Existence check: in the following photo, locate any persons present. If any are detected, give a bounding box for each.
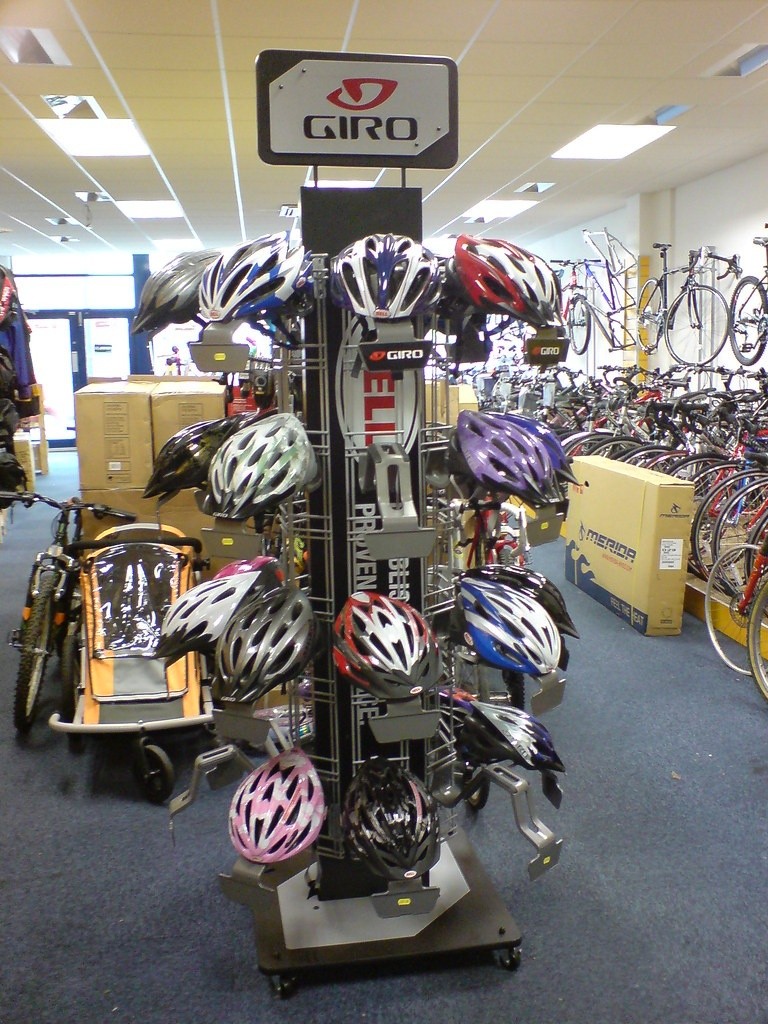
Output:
[163,346,185,377]
[165,358,175,376]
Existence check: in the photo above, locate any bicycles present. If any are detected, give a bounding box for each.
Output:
[462,233,768,703]
[0,489,140,756]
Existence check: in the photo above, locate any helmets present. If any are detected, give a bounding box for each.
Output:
[451,409,555,498]
[437,689,478,728]
[329,233,443,322]
[332,591,443,699]
[426,255,488,338]
[130,249,223,331]
[486,411,579,488]
[215,584,320,705]
[453,234,564,330]
[213,555,281,585]
[470,701,568,773]
[228,747,326,864]
[460,575,561,675]
[464,563,580,640]
[336,755,440,879]
[206,412,319,521]
[198,231,315,323]
[141,412,246,507]
[149,570,264,668]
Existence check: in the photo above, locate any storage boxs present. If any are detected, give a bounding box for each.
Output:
[80,489,158,561]
[425,379,459,434]
[73,381,158,491]
[159,489,257,582]
[13,431,35,492]
[459,383,479,411]
[565,455,695,638]
[151,381,226,489]
[227,386,274,416]
